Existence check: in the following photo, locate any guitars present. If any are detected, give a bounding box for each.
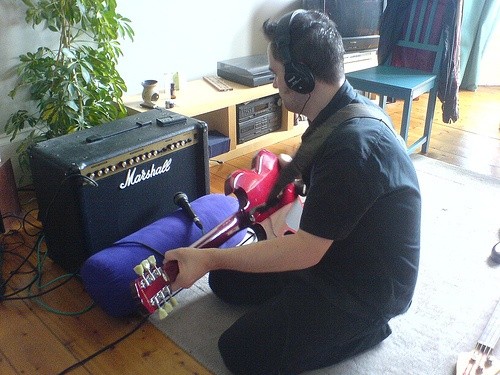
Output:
[129,149,303,320]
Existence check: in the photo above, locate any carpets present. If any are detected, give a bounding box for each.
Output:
[148,152,500,374]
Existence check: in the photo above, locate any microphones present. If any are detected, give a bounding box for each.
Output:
[174,191,205,231]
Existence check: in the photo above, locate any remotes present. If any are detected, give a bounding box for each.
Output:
[156,114,187,127]
[202,74,233,91]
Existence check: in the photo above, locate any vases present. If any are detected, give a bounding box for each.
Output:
[140,80,161,107]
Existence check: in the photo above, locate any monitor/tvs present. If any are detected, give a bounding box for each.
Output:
[301,0,387,51]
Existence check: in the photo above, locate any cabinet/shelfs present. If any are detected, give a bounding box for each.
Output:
[118,47,380,170]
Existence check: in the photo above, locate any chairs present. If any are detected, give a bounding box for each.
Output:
[342,1,465,160]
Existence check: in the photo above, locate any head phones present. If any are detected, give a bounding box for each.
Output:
[274,8,317,94]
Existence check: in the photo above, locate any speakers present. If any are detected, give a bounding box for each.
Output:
[29,106,212,273]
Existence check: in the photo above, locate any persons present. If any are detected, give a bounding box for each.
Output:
[162,9,421,373]
